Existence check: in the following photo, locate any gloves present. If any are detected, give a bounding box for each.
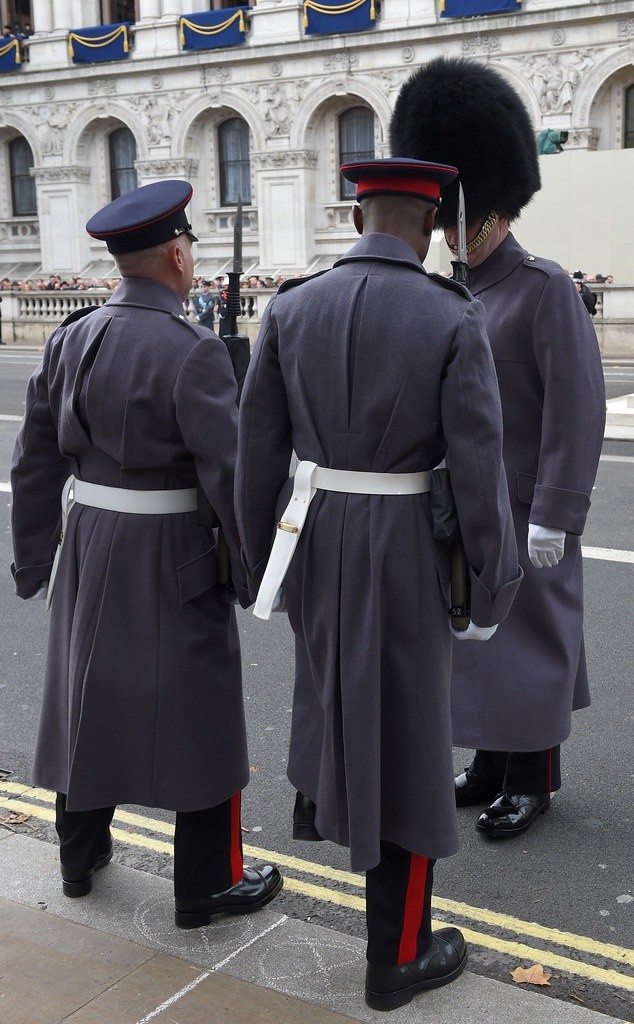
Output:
[32,581,48,599]
[271,588,287,614]
[527,523,566,569]
[448,618,500,642]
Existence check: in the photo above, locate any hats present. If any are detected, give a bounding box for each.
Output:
[86,179,199,253]
[201,281,212,287]
[338,156,460,209]
[388,53,540,228]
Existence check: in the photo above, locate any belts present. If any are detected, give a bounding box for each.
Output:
[41,473,196,614]
[253,452,435,620]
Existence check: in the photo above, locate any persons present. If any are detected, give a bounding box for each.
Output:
[0,274,122,316]
[0,296,6,346]
[185,275,288,318]
[192,280,215,332]
[217,288,229,340]
[435,165,608,843]
[0,20,34,64]
[10,179,284,929]
[231,155,526,1014]
[565,270,614,319]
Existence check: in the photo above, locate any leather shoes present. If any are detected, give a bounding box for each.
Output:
[173,863,284,929]
[60,833,114,897]
[292,789,322,842]
[475,792,552,838]
[453,767,499,808]
[365,927,471,1010]
